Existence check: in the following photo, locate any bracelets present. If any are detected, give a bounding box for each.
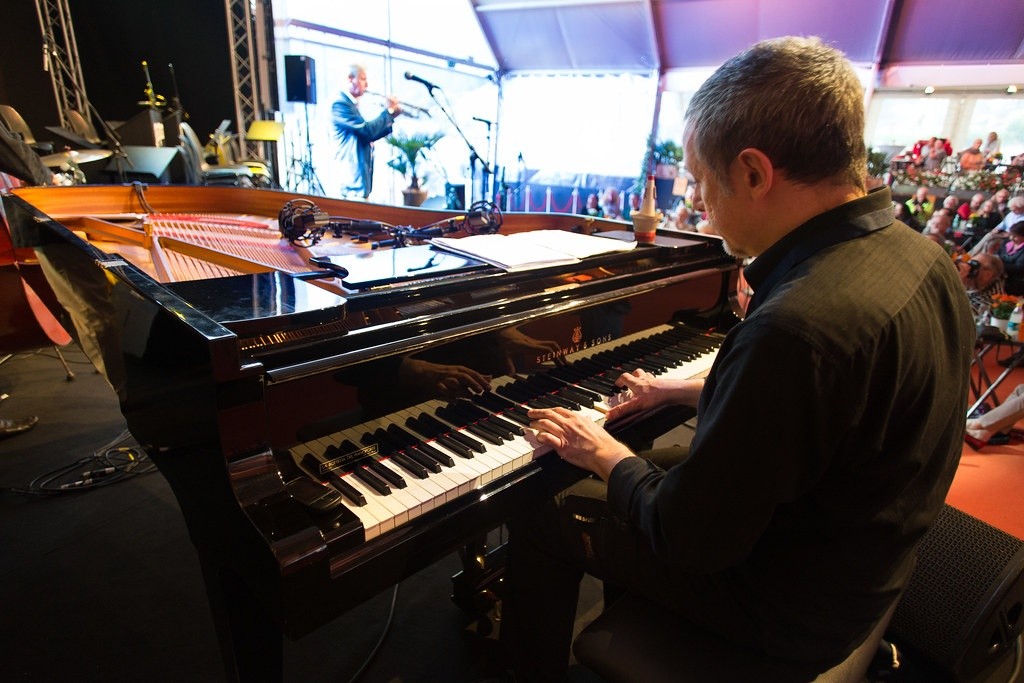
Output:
[388,107,394,115]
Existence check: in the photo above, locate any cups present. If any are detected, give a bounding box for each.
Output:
[631,214,658,243]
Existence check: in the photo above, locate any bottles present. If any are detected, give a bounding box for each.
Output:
[643,176,656,213]
[1006,303,1023,336]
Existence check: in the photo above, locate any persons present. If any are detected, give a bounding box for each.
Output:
[581,186,723,236]
[492,32,976,683]
[866,132,1024,450]
[328,64,403,202]
[403,328,570,398]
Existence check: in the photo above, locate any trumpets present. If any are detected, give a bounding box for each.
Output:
[366,90,431,119]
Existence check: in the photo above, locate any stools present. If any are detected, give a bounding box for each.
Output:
[572,557,920,683]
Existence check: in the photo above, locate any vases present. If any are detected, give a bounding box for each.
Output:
[990,316,1010,340]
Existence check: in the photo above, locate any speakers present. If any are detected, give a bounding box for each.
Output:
[285,54,317,104]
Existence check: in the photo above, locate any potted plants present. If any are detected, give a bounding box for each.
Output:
[865,147,891,192]
[647,139,683,181]
[383,130,446,207]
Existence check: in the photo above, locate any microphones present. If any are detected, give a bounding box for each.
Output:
[82,465,126,478]
[60,477,102,489]
[473,117,493,124]
[518,152,522,162]
[43,34,49,71]
[404,71,440,89]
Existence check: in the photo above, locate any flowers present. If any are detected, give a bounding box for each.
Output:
[892,163,1024,194]
[991,295,1018,320]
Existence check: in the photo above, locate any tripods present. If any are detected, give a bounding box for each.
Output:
[294,103,326,196]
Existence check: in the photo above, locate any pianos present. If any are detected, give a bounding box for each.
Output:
[0,179,748,683]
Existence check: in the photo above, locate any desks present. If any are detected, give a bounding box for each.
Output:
[966,334,1023,419]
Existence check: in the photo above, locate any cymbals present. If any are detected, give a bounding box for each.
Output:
[38,149,114,168]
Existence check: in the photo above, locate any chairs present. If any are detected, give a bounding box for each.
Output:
[175,122,273,190]
